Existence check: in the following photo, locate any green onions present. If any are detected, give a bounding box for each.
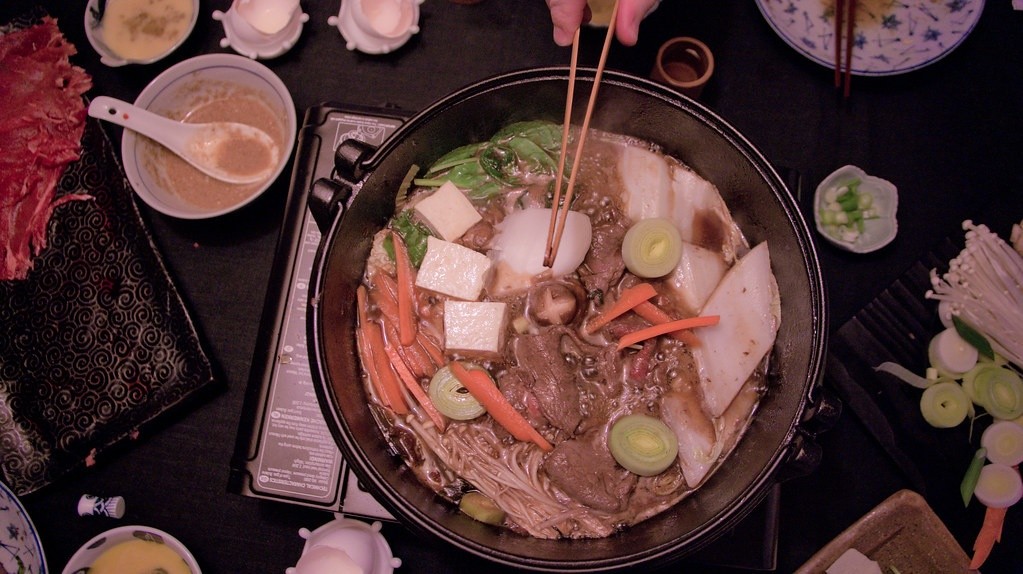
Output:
[872,327,1023,508]
[820,177,882,244]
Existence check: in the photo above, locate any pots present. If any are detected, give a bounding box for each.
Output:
[309,64,830,574]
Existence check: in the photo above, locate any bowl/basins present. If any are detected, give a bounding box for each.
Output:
[122,52,298,220]
[814,165,898,254]
[84,0,200,68]
[61,524,202,574]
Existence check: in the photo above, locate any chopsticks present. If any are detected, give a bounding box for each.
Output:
[542,0,620,268]
[831,0,855,96]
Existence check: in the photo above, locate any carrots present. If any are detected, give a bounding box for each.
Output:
[969,506,1007,569]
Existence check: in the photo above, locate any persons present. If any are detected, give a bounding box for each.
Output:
[546,0,1023,44]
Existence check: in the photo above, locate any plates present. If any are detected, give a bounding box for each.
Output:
[756,0,985,76]
[0,482,48,574]
[0,96,216,497]
[795,489,980,574]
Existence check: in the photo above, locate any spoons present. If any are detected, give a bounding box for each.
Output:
[86,96,279,184]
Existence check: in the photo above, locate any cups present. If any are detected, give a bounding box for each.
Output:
[651,37,715,100]
